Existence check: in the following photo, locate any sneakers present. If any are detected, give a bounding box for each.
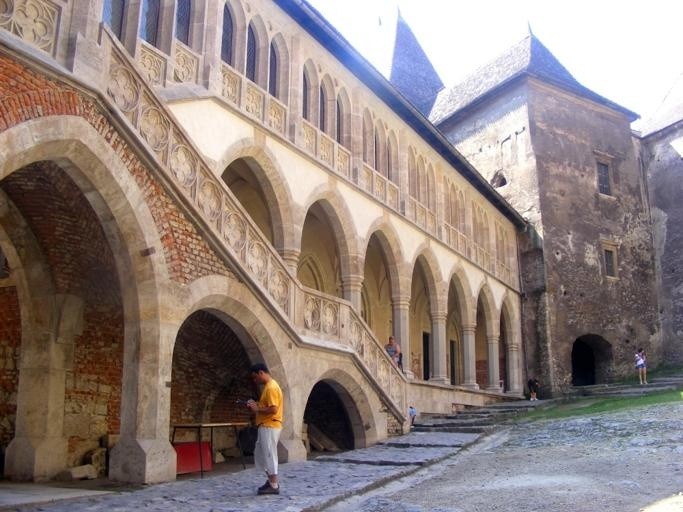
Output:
[258,481,279,495]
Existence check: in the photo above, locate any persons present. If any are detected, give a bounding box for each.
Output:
[528,376,538,401]
[633,348,648,385]
[247,364,282,494]
[384,336,400,366]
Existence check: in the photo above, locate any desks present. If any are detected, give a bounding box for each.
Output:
[171,421,252,477]
